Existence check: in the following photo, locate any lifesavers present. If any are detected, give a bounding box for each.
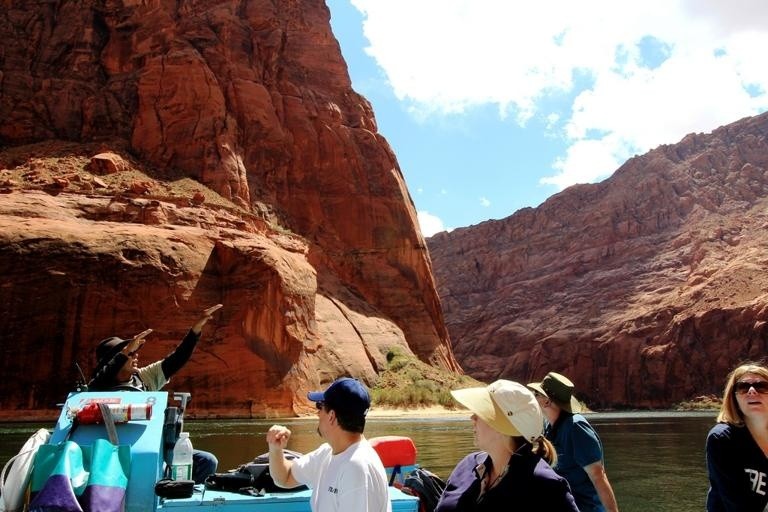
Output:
[0,427,51,510]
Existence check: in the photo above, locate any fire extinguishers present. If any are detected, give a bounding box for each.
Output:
[65,399,153,424]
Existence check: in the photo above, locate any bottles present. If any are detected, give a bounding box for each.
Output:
[70,403,153,425]
[171,431,193,481]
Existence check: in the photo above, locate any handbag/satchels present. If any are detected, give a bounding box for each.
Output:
[28,439,132,512]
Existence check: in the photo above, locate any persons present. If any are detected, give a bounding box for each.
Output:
[433,378,580,512]
[526,372,620,512]
[706,364,768,512]
[88,303,224,483]
[266,377,392,512]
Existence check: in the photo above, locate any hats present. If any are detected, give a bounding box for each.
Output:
[308,377,370,417]
[89,337,144,378]
[527,372,582,414]
[450,378,543,444]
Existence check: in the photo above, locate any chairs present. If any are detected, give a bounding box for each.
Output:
[369,437,419,486]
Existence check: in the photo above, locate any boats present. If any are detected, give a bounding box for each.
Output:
[0,389,426,512]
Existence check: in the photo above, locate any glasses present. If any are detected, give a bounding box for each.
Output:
[316,402,327,410]
[734,381,768,395]
